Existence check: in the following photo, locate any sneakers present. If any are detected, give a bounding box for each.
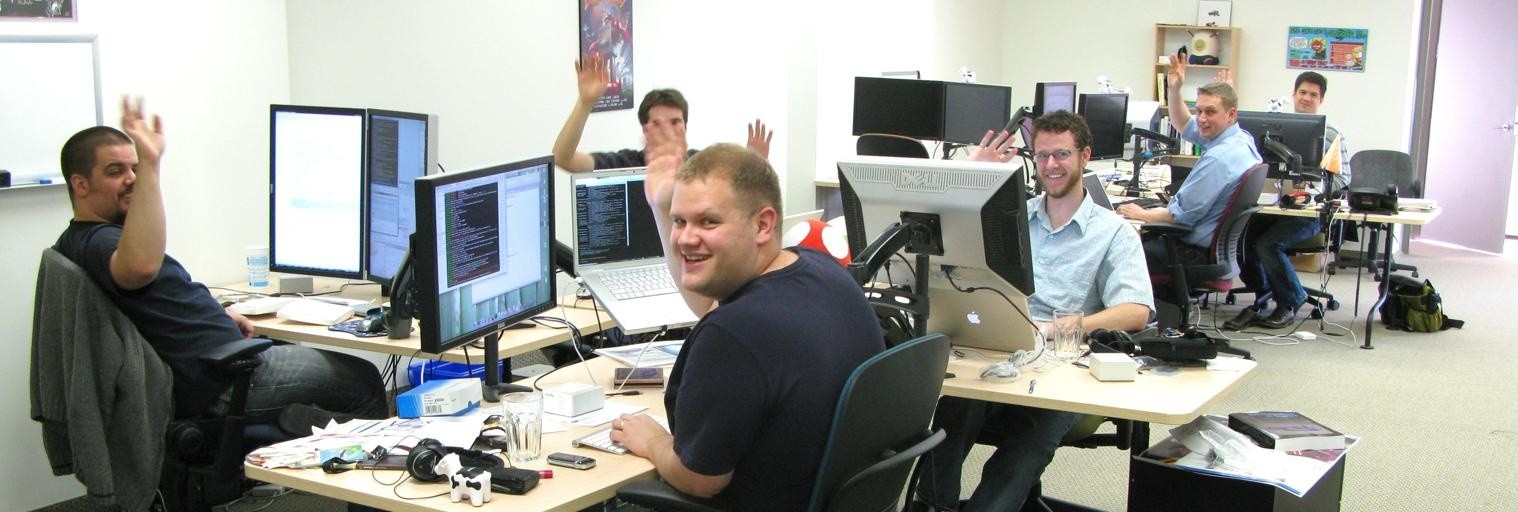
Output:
[278,402,378,436]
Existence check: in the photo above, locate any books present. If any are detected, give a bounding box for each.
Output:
[1228,411,1346,452]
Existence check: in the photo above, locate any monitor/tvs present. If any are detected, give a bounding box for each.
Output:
[1078,93,1129,161]
[414,154,558,403]
[269,104,367,280]
[944,82,1012,146]
[1117,101,1161,162]
[1236,111,1326,182]
[365,107,440,287]
[1033,81,1076,119]
[835,155,1036,296]
[572,413,671,456]
[852,76,945,141]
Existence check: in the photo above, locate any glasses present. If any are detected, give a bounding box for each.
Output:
[1032,147,1084,163]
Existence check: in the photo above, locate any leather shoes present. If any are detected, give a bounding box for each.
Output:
[1223,306,1262,331]
[1259,304,1294,328]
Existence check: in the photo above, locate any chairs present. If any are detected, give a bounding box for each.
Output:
[1328,150,1423,281]
[857,133,929,158]
[1143,163,1270,335]
[936,393,1134,510]
[29,249,289,512]
[616,334,951,512]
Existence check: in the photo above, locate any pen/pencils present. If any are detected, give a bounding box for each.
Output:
[538,470,553,479]
[1029,379,1036,393]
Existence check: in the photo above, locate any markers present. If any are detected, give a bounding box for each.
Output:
[40,178,67,184]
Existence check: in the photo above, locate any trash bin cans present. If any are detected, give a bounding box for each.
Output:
[407,359,504,388]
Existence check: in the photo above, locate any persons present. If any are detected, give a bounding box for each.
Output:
[913,108,1159,512]
[1114,52,1265,277]
[592,142,887,512]
[52,93,384,512]
[551,51,774,172]
[1223,71,1352,331]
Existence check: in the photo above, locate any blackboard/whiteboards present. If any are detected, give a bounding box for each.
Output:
[0,36,104,190]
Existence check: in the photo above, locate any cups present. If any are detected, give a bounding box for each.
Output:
[246,246,269,289]
[504,389,543,463]
[1052,307,1084,357]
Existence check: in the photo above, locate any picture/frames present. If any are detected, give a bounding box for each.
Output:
[1197,0,1232,26]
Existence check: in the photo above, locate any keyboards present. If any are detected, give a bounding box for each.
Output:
[1257,192,1278,205]
[1112,198,1163,211]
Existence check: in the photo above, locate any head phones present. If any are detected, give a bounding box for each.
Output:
[406,438,505,483]
[1089,328,1135,354]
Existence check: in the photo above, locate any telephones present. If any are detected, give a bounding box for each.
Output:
[1347,184,1399,213]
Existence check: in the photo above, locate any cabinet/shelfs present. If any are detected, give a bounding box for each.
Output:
[1152,25,1240,110]
[1128,414,1346,512]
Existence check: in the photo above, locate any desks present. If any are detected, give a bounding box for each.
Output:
[244,339,685,512]
[814,173,1171,223]
[208,271,616,383]
[1106,164,1441,350]
[863,283,1257,456]
[556,290,602,310]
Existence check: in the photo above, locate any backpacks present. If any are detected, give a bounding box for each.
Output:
[1377,273,1444,333]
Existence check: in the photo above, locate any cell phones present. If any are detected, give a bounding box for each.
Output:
[547,453,596,470]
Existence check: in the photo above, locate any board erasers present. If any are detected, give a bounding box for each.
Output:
[0,169,11,188]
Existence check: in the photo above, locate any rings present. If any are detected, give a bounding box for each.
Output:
[621,419,625,429]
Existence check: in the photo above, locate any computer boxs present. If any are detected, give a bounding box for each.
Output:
[1127,436,1346,512]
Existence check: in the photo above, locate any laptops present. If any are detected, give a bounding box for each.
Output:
[570,166,700,337]
[925,288,1054,353]
[1082,171,1116,213]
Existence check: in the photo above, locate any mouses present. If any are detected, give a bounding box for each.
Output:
[357,315,386,333]
